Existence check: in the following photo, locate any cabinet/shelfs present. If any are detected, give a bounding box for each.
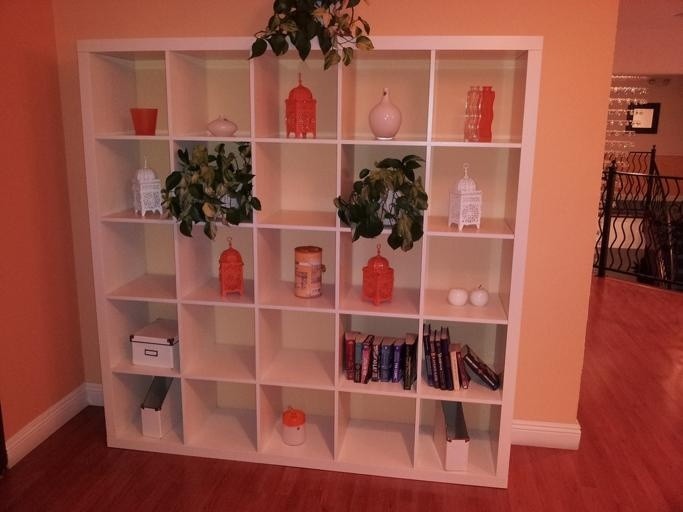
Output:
[72,37,545,491]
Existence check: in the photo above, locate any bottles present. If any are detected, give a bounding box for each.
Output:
[284,409,306,446]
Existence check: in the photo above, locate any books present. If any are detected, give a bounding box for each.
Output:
[423,324,501,394]
[344,326,418,390]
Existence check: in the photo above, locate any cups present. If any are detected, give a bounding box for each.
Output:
[131,108,159,135]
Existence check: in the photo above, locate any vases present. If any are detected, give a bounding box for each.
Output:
[465,84,496,142]
[369,86,402,140]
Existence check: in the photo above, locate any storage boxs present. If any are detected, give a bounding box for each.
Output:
[130,317,179,368]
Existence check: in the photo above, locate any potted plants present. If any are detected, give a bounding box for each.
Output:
[335,156,432,253]
[160,140,262,240]
[246,0,376,70]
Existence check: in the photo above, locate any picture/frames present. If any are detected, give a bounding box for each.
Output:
[625,101,661,134]
